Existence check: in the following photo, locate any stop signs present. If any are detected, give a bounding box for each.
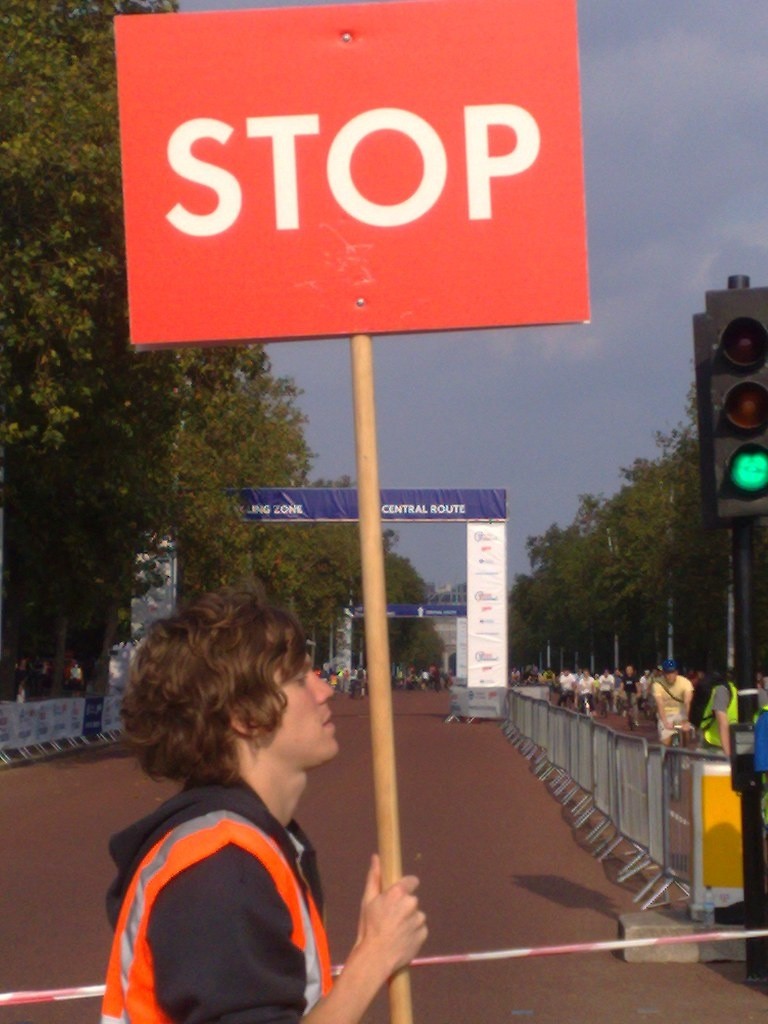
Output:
[107,0,593,343]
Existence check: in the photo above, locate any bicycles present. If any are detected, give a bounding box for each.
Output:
[564,686,656,731]
[668,722,697,800]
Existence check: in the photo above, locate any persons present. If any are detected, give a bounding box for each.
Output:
[505,651,744,759]
[315,660,453,693]
[10,649,86,702]
[101,581,428,1024]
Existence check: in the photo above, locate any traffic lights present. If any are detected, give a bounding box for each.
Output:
[701,288,768,521]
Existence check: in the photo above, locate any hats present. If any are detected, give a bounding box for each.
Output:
[662,659,677,673]
